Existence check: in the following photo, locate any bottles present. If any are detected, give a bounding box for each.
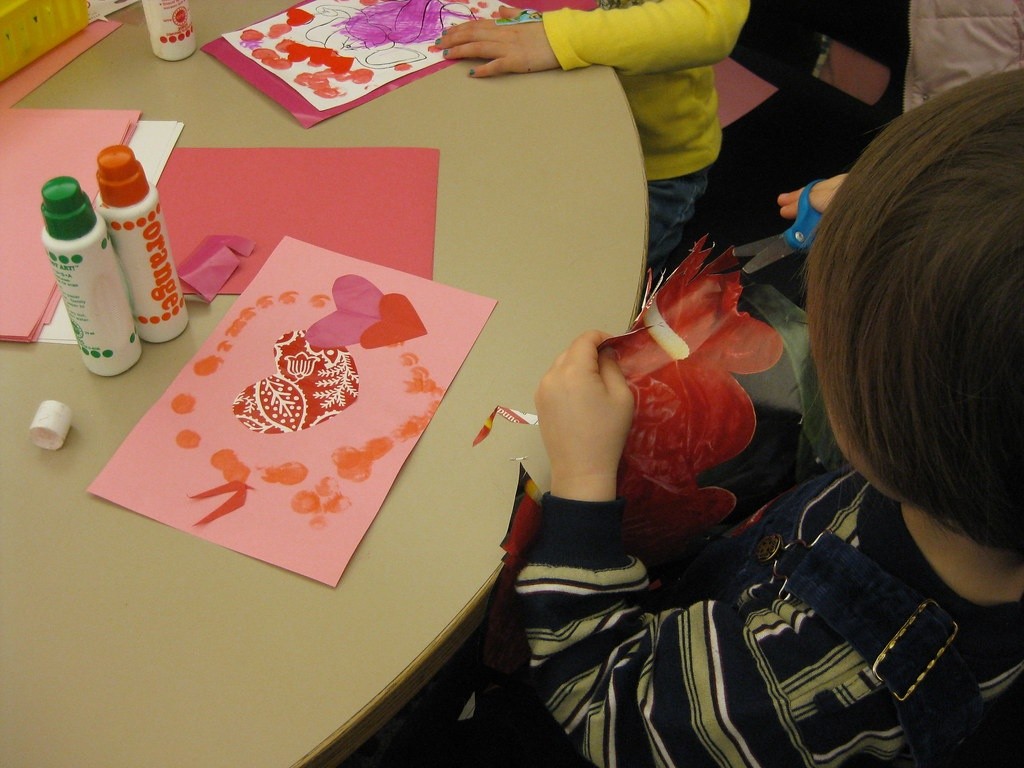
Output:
[142,0,197,62]
[40,176,143,378]
[91,146,188,344]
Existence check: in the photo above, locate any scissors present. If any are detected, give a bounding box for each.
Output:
[731,178,855,275]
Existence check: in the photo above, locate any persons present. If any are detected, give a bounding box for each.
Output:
[511,70,1024,767]
[435,0,751,268]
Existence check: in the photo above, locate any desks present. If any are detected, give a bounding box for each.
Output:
[1,1,650,768]
